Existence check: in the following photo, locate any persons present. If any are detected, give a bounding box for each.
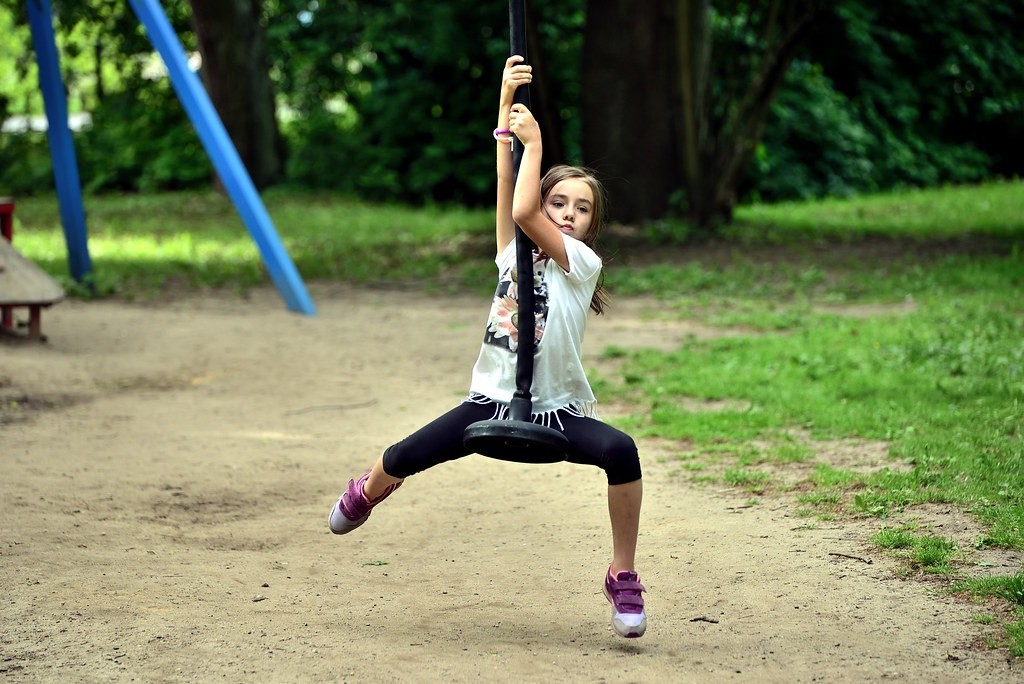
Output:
[324,54,649,640]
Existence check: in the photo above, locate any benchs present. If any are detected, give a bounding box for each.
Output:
[0,230,67,340]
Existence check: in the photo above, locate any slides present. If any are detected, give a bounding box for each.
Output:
[0,231,65,307]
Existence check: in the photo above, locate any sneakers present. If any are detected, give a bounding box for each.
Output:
[328,470,404,534]
[601,564,647,637]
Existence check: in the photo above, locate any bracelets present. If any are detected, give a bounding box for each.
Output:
[493,127,514,144]
